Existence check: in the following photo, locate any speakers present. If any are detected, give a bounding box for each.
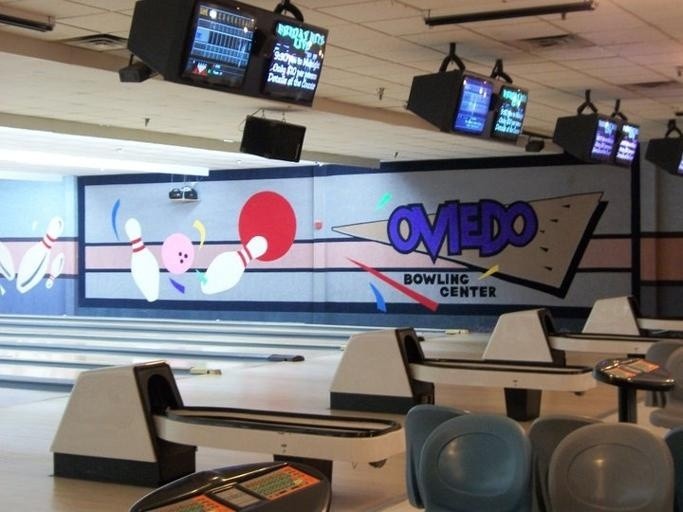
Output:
[239,115,306,163]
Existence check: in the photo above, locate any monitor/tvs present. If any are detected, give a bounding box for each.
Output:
[127,0,258,90]
[253,8,329,108]
[406,69,494,135]
[490,84,528,146]
[553,112,619,163]
[608,123,641,169]
[644,137,683,177]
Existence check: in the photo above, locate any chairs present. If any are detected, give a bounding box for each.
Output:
[405,404,683,510]
[643,339,682,429]
